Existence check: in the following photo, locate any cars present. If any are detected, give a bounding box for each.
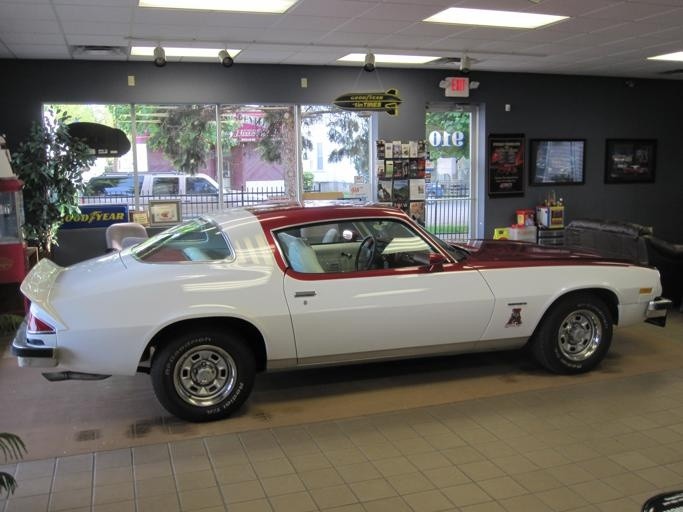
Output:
[612,155,648,178]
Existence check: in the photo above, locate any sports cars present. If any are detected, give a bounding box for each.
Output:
[12,202,667,422]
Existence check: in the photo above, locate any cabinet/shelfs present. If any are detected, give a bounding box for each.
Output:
[377,158,426,227]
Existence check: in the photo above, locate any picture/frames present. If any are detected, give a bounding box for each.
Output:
[603,137,658,184]
[148,199,183,227]
[130,212,150,227]
[528,138,586,187]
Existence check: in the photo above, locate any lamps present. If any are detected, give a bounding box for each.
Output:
[153,42,167,68]
[459,52,476,73]
[216,44,235,69]
[363,46,377,71]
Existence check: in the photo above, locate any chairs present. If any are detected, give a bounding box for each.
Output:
[277,231,325,274]
[182,245,212,262]
[105,222,148,251]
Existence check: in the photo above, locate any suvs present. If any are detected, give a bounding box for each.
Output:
[78,170,265,226]
[424,179,444,205]
